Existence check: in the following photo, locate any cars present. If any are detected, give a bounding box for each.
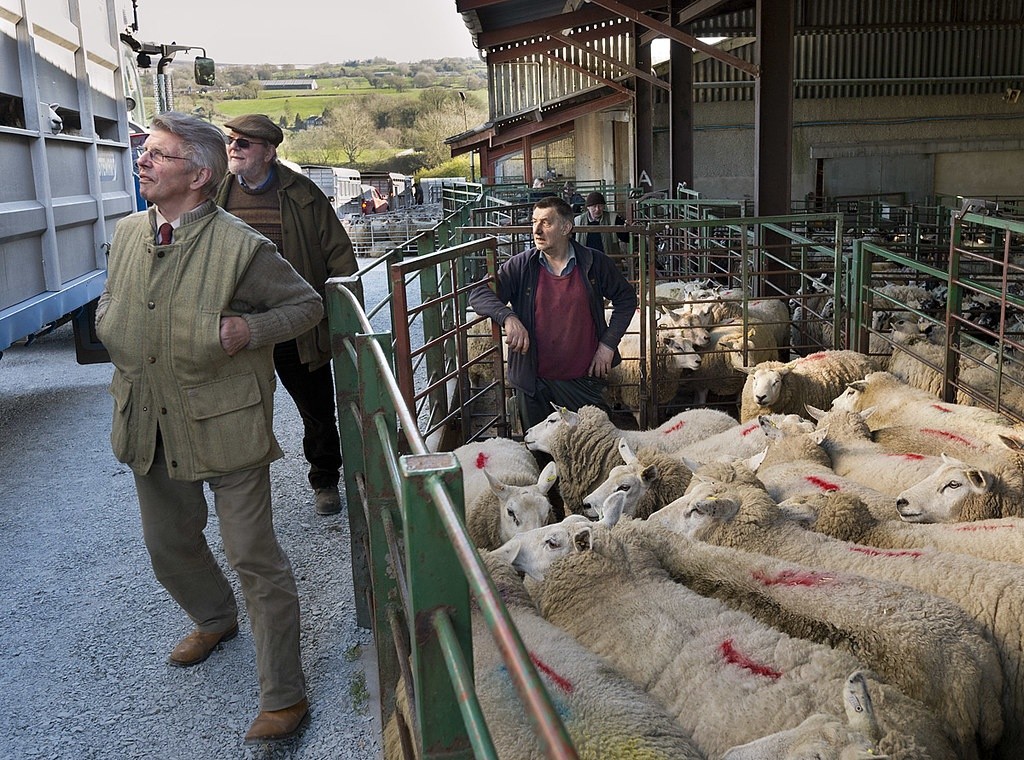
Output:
[361,184,390,214]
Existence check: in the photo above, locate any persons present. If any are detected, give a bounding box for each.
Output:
[572,192,633,276]
[211,113,364,516]
[96,110,328,743]
[467,193,638,518]
[557,180,586,214]
[522,178,558,211]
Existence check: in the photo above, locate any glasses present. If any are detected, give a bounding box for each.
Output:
[224,135,264,149]
[134,149,192,163]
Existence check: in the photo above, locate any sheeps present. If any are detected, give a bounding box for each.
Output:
[384,223,1024,760]
[336,202,441,259]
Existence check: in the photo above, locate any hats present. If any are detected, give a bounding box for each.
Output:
[585,192,606,208]
[223,114,283,143]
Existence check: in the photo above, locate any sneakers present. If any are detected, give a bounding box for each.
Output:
[314,486,341,515]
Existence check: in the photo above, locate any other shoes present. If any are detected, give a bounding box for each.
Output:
[167,620,240,668]
[243,695,310,746]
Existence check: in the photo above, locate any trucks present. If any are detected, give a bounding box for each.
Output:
[0,0,215,363]
